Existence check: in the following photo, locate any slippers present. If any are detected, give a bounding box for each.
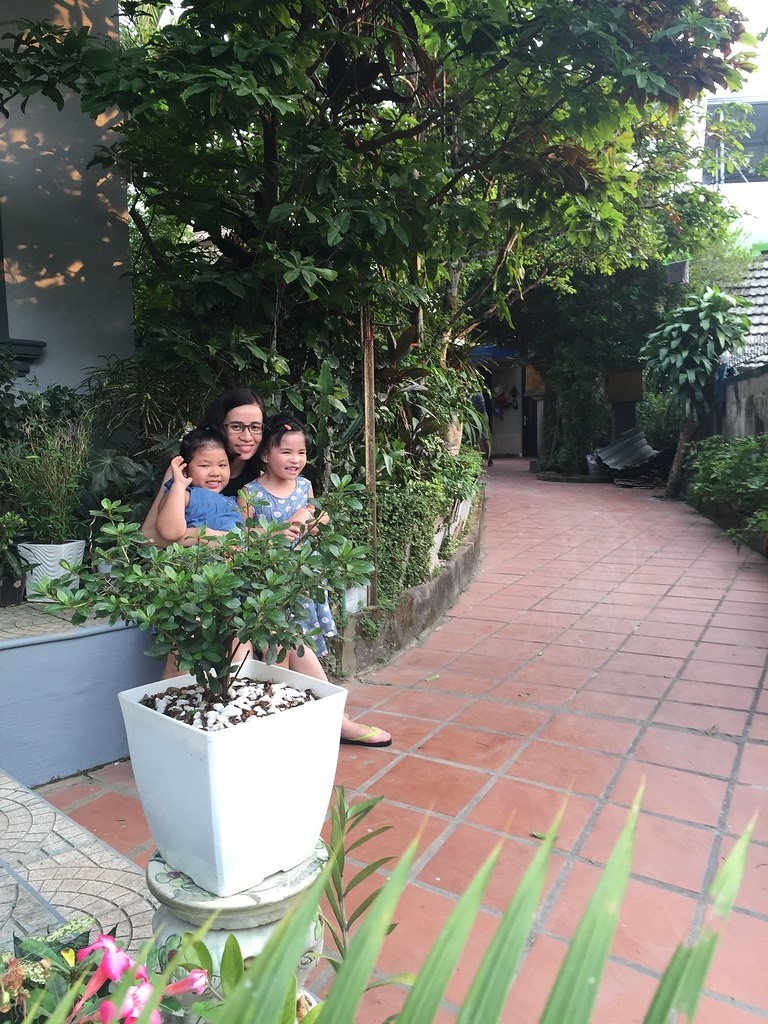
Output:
[339,722,392,747]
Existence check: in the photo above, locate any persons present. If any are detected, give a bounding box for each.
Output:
[139,387,392,749]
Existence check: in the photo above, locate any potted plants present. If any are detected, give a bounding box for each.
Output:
[0,391,126,603]
[338,384,493,613]
[24,473,374,896]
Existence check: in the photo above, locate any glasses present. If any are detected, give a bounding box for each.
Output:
[222,421,265,434]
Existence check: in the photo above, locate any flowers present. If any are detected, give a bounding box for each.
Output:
[0,933,211,1024]
